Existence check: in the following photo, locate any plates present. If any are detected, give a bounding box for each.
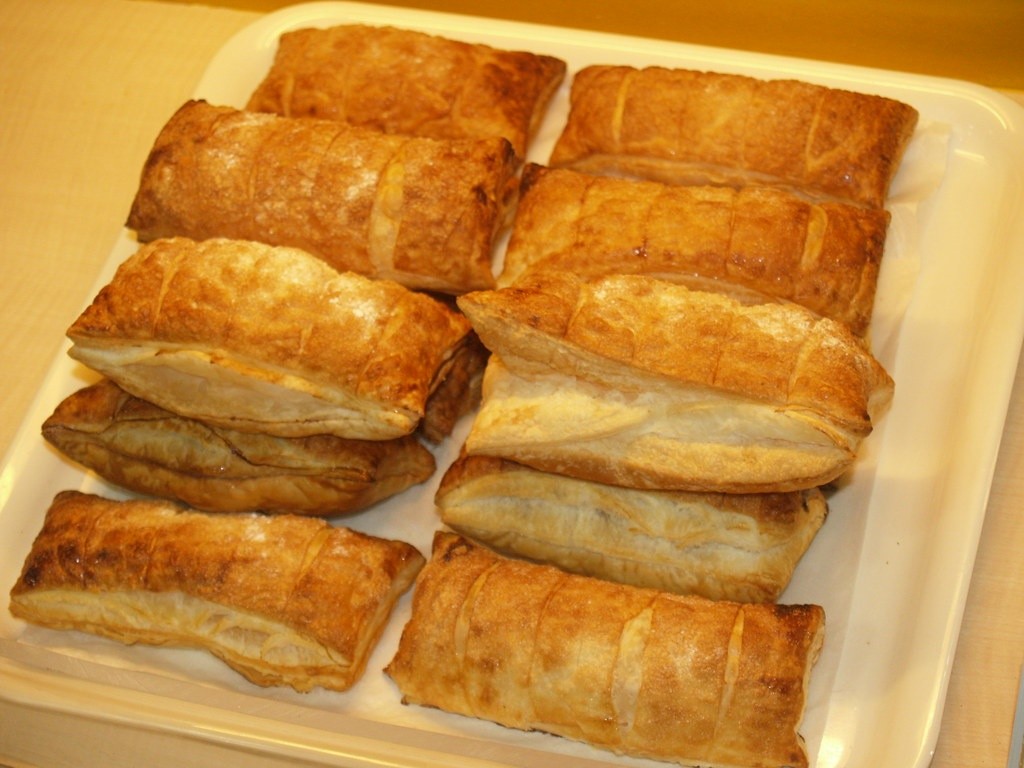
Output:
[0,0,1024,768]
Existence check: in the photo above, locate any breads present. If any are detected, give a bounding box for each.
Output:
[13,27,914,768]
